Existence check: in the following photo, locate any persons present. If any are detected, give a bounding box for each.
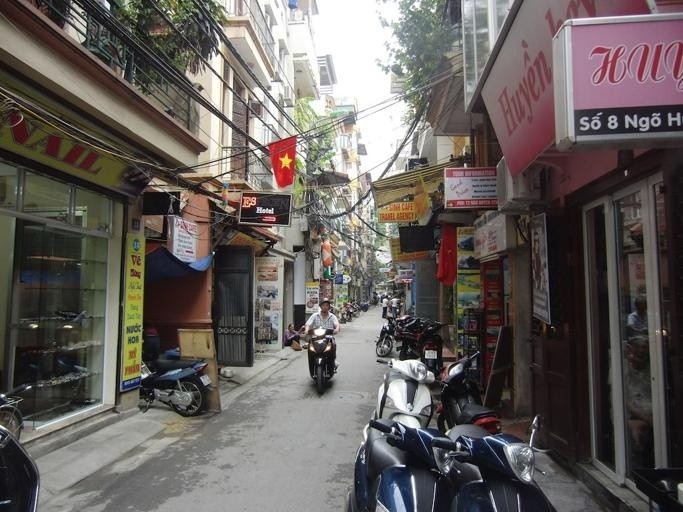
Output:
[374,293,379,307]
[390,295,400,319]
[380,294,388,318]
[606,334,654,453]
[302,297,340,373]
[380,293,385,305]
[283,323,305,347]
[627,291,648,337]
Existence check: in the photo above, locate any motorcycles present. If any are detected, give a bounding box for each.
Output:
[0,382,41,511]
[330,295,377,324]
[619,332,656,459]
[302,324,335,396]
[137,358,211,416]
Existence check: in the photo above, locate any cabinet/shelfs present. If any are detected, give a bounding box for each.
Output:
[4,223,107,432]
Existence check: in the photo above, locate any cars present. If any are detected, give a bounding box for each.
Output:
[380,291,392,302]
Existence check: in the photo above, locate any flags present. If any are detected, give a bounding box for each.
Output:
[265,135,298,190]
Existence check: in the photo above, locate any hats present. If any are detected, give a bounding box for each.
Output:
[319,297,331,307]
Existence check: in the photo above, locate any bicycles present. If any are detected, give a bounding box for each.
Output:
[0,396,24,441]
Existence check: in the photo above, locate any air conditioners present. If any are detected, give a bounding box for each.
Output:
[495,154,545,216]
